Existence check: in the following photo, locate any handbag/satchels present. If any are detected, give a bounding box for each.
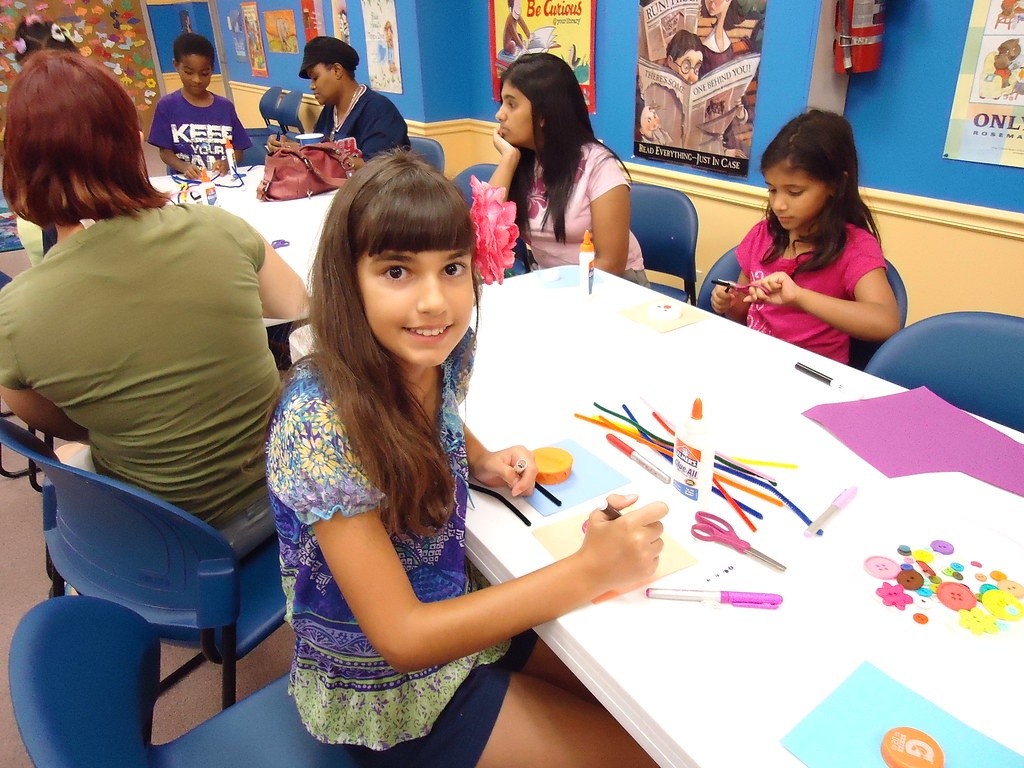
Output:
[256,142,356,202]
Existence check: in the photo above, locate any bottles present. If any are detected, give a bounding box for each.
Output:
[579,228,595,294]
[671,398,715,511]
[199,170,220,209]
[224,137,238,179]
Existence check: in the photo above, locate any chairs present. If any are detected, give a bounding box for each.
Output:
[0,87,1024,768]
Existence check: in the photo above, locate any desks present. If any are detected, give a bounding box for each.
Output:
[289,265,1024,768]
[104,165,340,327]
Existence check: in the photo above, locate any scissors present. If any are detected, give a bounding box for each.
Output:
[271,240,290,249]
[690,511,787,572]
[717,278,770,305]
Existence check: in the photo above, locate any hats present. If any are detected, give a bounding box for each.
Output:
[299,36,359,79]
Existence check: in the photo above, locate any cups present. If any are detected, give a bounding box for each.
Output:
[295,133,324,144]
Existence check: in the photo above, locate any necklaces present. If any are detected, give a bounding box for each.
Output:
[328,86,363,141]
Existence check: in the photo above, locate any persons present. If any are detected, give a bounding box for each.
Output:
[0,49,312,596]
[14,17,144,266]
[488,52,650,289]
[148,33,253,181]
[0,271,13,289]
[267,36,412,163]
[267,148,669,768]
[710,109,901,365]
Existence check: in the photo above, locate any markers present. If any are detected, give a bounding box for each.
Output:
[597,498,625,520]
[804,486,859,538]
[795,363,864,399]
[606,434,671,484]
[646,588,784,609]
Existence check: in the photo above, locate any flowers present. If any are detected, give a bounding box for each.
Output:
[470,174,520,285]
